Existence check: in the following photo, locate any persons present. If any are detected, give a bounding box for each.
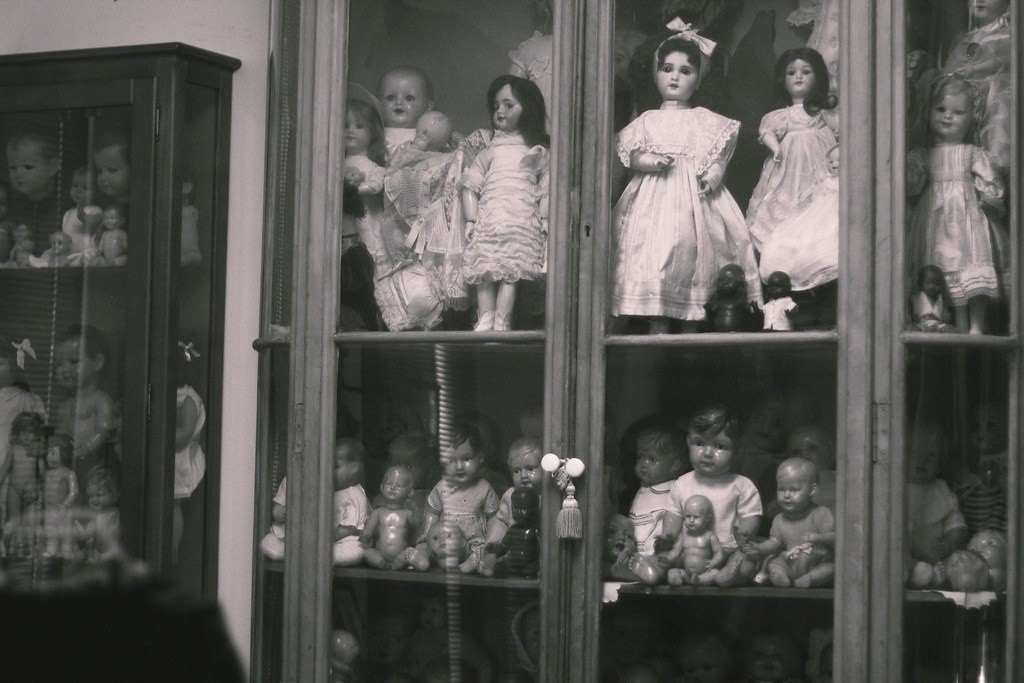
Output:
[0,124,206,569]
[337,33,554,332]
[262,404,1009,683]
[608,16,839,330]
[904,0,1011,338]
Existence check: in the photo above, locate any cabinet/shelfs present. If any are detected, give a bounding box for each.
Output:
[250,0,1024,683]
[0,41,247,683]
